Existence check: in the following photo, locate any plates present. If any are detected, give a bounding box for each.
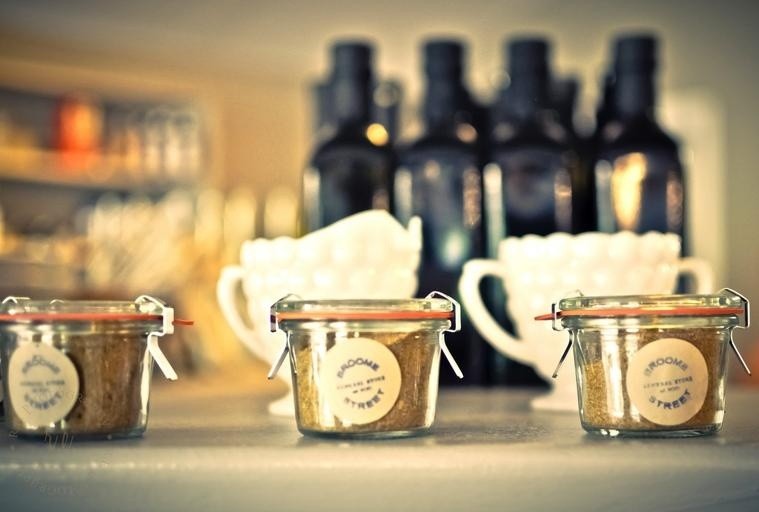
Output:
[1,61,219,273]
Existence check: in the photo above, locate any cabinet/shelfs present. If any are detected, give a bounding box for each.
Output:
[393,39,483,392]
[483,39,587,388]
[588,34,682,292]
[298,40,393,298]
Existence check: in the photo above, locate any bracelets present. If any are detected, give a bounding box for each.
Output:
[2,296,171,438]
[266,296,455,438]
[558,294,743,438]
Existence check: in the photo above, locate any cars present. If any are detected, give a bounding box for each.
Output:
[213,236,424,418]
[459,229,713,416]
